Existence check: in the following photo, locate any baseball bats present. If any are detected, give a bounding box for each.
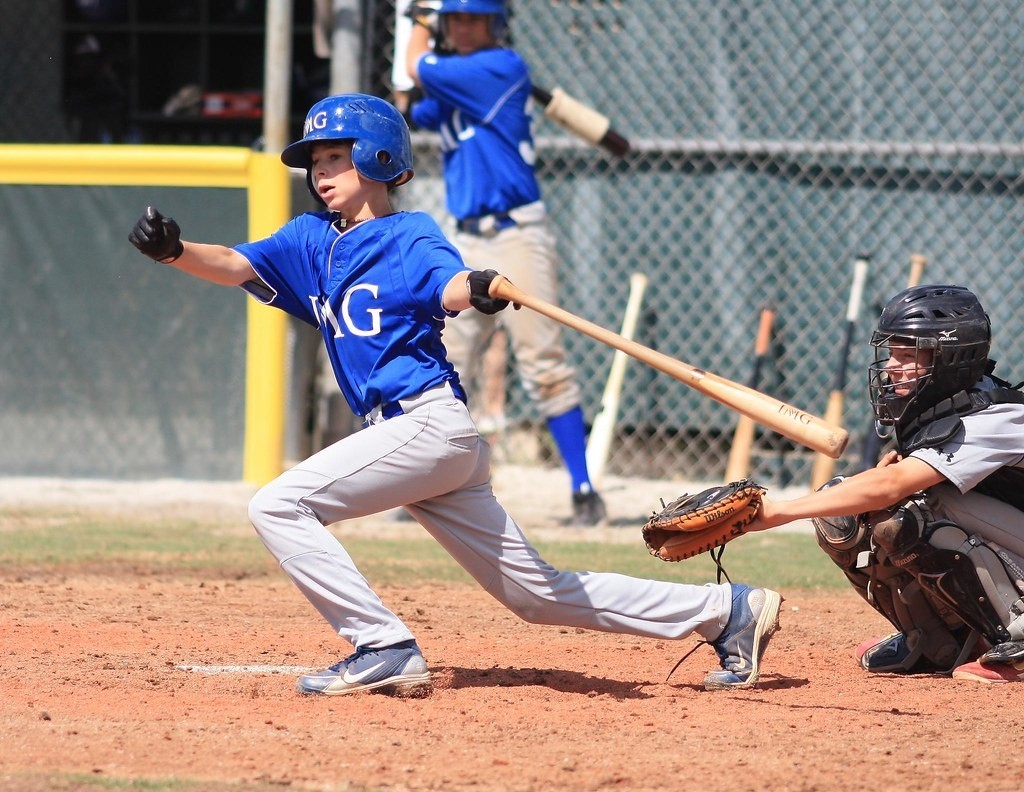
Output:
[721,308,773,487]
[584,272,653,478]
[807,255,930,488]
[488,273,851,465]
[402,5,632,167]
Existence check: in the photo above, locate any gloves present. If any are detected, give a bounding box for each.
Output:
[403,0,444,35]
[466,268,522,315]
[128,206,184,265]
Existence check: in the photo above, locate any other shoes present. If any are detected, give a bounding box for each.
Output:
[376,502,419,523]
[567,490,607,530]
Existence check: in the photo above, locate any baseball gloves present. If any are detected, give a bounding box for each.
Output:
[638,477,768,568]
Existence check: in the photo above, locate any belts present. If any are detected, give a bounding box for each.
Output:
[362,380,467,427]
[456,210,517,236]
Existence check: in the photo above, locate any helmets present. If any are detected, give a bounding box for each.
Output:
[867,282,991,442]
[439,0,512,49]
[281,93,415,205]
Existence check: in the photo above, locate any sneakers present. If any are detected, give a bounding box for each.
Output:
[663,581,787,691]
[861,631,931,672]
[951,649,1024,684]
[295,639,436,695]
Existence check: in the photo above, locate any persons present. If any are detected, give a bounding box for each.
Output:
[737,284,1023,689]
[391,0,609,527]
[128,95,780,698]
